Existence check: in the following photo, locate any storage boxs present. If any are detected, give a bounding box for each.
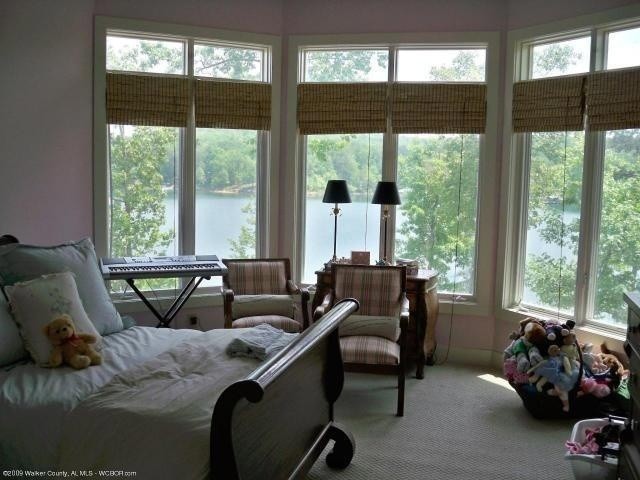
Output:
[564,418,625,479]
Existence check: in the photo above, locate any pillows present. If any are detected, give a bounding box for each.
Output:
[3,237,124,336]
[339,314,402,342]
[0,288,29,366]
[231,293,295,320]
[5,271,108,368]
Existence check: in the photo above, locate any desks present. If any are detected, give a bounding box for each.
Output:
[313,270,440,379]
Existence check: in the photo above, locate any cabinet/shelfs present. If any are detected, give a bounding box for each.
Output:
[617,291,640,480]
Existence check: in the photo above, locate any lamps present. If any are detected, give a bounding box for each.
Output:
[322,180,353,270]
[370,181,401,265]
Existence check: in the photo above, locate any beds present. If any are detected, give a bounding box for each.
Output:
[0,235,360,480]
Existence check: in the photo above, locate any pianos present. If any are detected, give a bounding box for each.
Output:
[99,255,228,280]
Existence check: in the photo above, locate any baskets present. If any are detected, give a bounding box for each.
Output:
[505,322,596,419]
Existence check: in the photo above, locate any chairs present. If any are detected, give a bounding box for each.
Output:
[313,261,411,416]
[219,257,311,333]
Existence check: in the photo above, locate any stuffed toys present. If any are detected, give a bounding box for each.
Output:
[43,314,103,370]
[502,315,630,412]
